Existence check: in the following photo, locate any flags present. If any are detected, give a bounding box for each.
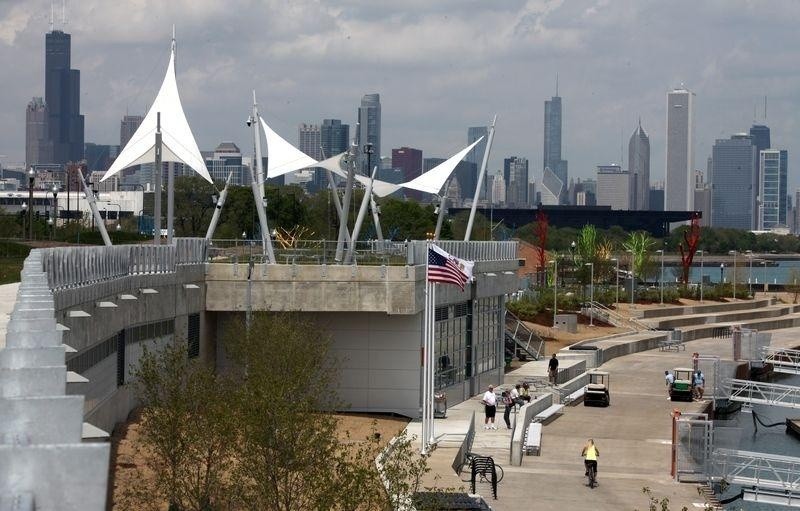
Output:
[428,248,465,292]
[432,244,472,284]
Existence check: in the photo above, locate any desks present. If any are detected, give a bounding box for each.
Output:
[289,248,315,265]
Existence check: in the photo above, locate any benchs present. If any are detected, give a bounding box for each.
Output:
[535,404,565,423]
[526,423,543,456]
[309,255,324,265]
[279,253,304,264]
[565,387,584,405]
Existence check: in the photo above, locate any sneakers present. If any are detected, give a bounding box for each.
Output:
[484,425,497,429]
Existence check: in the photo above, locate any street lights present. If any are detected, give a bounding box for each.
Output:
[549,239,769,328]
[21,163,153,245]
[364,141,374,240]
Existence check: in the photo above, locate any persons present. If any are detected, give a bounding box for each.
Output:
[694,353,699,369]
[480,381,532,430]
[582,438,599,483]
[692,368,705,402]
[547,353,558,386]
[506,339,514,369]
[438,351,451,385]
[664,370,674,400]
[691,351,695,370]
